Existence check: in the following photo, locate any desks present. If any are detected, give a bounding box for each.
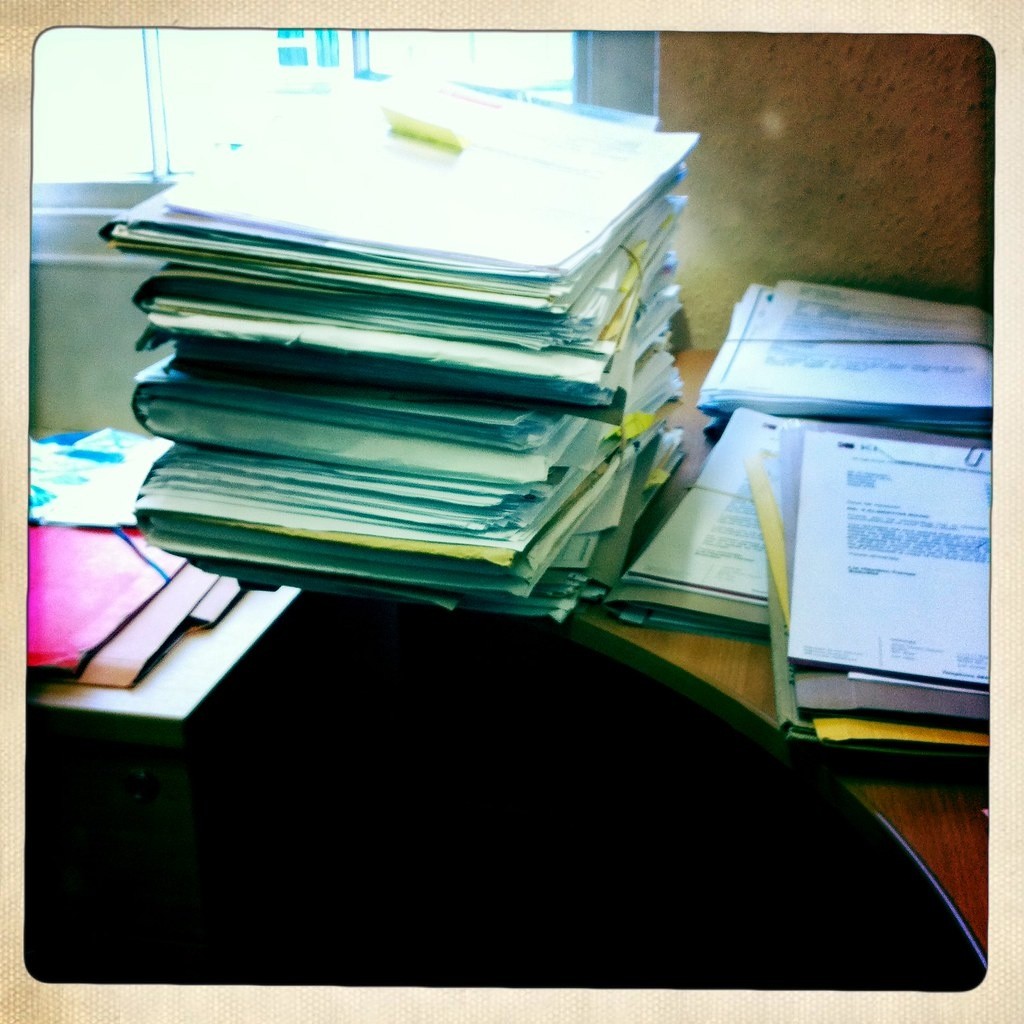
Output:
[572,348,993,967]
